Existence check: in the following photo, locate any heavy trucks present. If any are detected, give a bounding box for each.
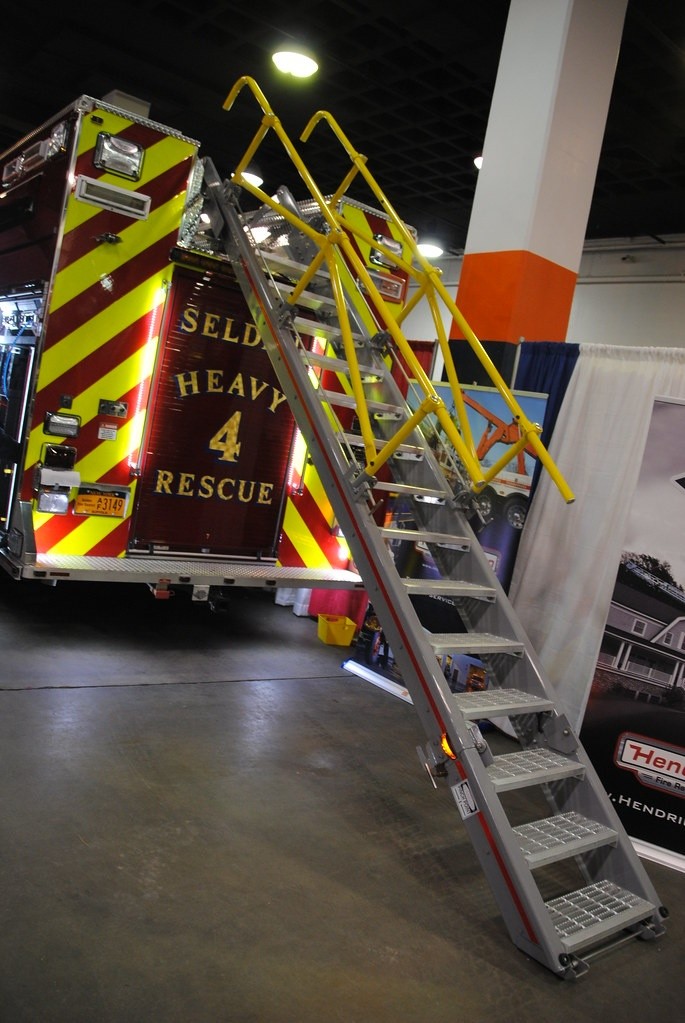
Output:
[438,443,532,530]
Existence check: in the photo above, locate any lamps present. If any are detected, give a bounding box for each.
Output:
[255,17,319,77]
[417,243,459,257]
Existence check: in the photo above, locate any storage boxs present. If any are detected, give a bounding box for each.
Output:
[318,614,358,647]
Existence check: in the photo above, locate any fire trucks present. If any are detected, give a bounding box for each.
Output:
[0,75,671,982]
